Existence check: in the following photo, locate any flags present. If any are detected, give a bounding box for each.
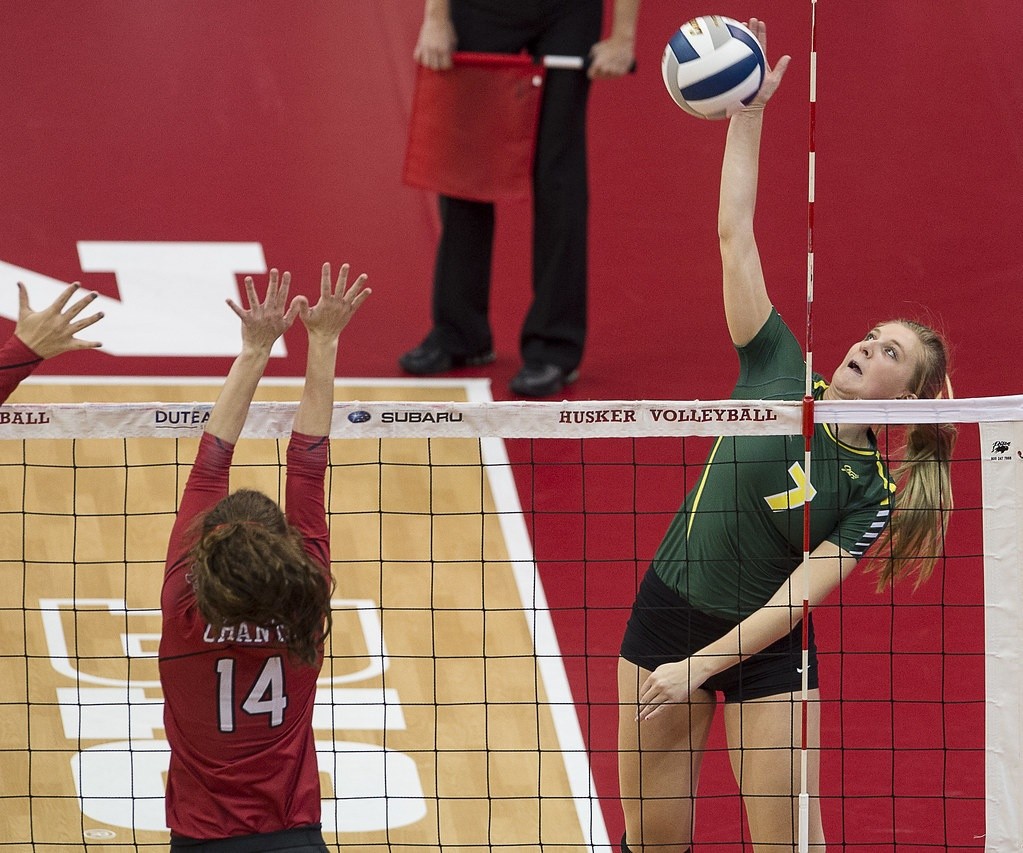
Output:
[402,53,546,204]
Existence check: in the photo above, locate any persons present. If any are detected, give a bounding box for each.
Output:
[618,15,954,853]
[398,0,642,397]
[159,262,373,853]
[0,281,104,408]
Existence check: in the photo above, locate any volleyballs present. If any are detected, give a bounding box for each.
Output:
[660,16,765,121]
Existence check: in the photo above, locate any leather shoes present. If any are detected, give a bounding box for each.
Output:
[397,331,494,374]
[509,362,579,397]
